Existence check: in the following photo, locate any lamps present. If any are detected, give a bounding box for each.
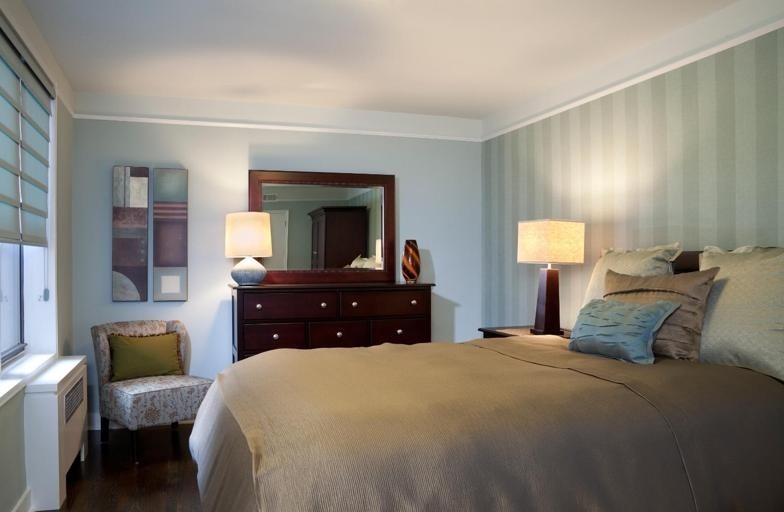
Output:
[516,218,585,334]
[375,238,382,263]
[223,208,275,285]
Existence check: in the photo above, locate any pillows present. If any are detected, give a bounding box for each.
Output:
[566,300,682,367]
[603,265,721,364]
[107,328,184,380]
[692,245,784,385]
[576,242,684,303]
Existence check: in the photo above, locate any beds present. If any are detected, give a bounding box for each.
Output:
[186,251,782,511]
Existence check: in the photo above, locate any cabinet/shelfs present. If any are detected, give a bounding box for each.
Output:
[231,281,436,365]
[308,206,368,270]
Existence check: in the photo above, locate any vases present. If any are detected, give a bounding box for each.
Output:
[401,239,421,287]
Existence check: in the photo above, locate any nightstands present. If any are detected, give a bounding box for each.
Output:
[478,325,575,344]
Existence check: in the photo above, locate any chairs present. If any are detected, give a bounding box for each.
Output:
[91,320,214,489]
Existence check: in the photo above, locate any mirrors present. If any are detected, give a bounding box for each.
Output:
[248,168,396,284]
